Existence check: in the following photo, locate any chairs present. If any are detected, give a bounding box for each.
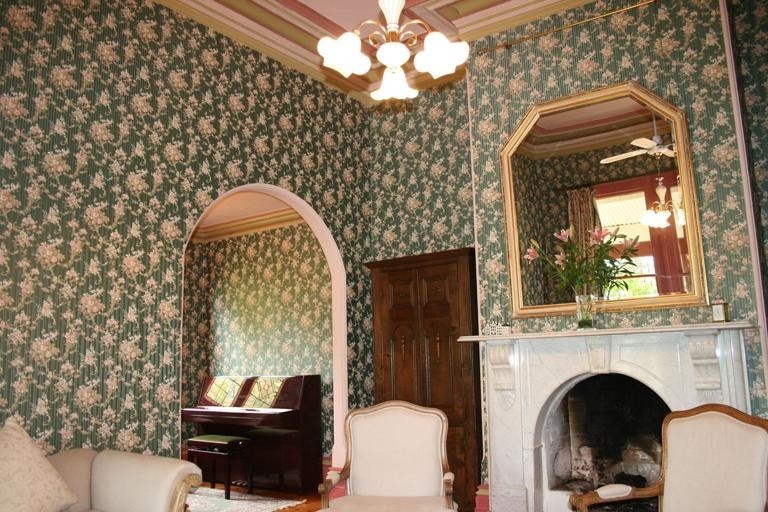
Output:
[569,404,768,512]
[315,400,458,512]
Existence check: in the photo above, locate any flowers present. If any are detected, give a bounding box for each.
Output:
[554,235,639,301]
[524,226,628,321]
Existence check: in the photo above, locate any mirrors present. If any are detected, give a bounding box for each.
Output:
[499,79,710,319]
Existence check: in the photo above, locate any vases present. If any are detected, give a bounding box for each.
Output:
[598,297,607,301]
[575,294,597,330]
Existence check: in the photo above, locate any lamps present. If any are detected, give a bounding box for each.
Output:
[317,0,470,101]
[641,159,686,229]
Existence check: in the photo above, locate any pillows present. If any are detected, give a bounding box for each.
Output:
[0,417,77,512]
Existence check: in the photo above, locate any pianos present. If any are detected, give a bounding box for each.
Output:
[181,374,323,500]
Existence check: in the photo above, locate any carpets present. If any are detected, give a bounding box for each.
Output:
[185,486,307,512]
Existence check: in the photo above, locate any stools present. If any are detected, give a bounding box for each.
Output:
[188,434,252,499]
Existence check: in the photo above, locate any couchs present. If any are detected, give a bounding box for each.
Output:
[47,448,203,512]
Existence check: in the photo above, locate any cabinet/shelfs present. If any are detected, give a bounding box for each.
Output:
[364,248,483,512]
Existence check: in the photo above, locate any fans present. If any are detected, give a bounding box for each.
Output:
[600,111,675,164]
